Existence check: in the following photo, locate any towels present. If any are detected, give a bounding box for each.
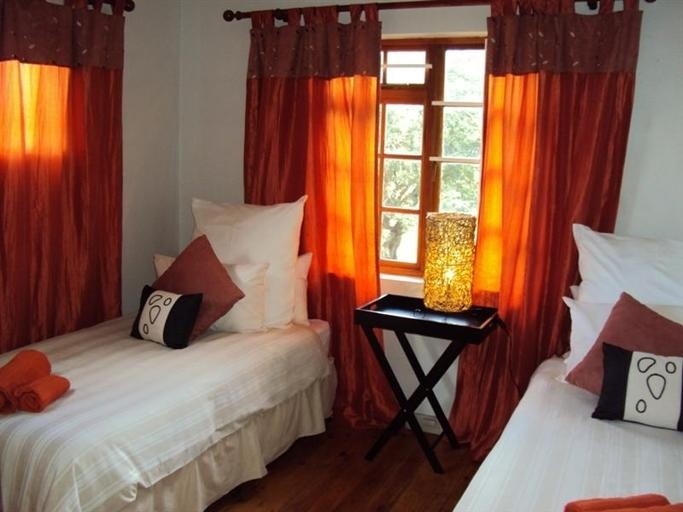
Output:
[14,376,70,411]
[0,350,51,412]
[595,502,682,512]
[563,494,670,512]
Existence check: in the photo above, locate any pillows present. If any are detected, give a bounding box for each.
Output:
[130,193,313,349]
[562,223,683,432]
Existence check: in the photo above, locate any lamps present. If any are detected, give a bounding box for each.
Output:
[423,212,477,313]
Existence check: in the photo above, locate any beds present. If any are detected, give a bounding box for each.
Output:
[0,310,338,512]
[450,355,683,512]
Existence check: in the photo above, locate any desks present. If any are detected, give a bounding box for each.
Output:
[354,294,498,474]
